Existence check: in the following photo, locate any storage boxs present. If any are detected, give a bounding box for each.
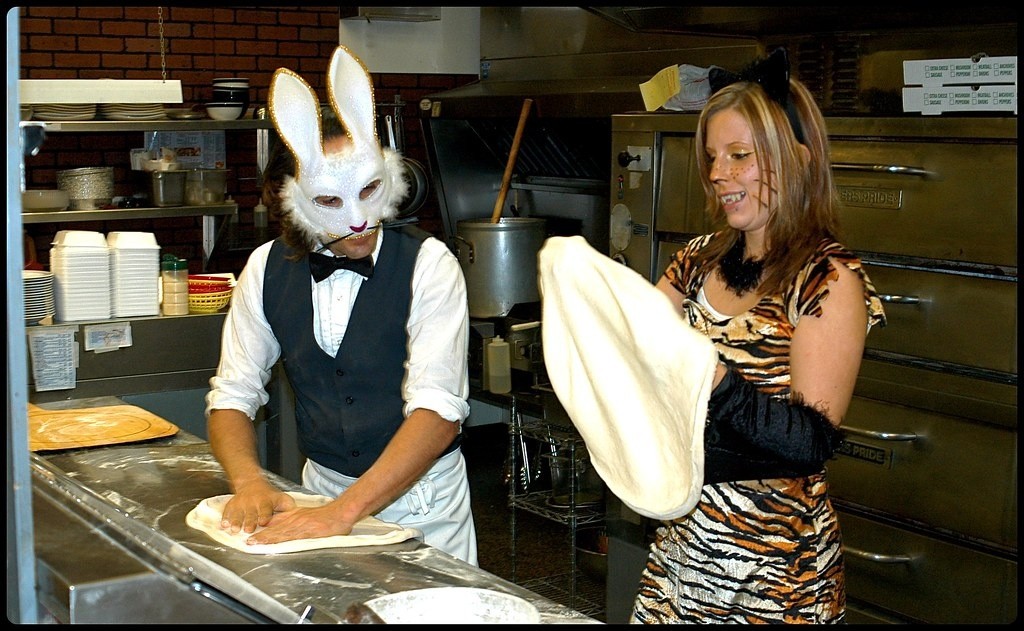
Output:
[901,56,1018,116]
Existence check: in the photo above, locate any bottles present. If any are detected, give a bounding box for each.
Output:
[162,254,189,315]
[224,195,268,248]
[487,335,511,394]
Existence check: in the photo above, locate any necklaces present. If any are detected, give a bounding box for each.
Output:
[718,231,774,298]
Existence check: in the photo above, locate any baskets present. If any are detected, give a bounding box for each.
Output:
[187,275,231,313]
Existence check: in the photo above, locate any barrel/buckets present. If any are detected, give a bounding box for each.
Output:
[449,218,546,319]
[541,450,604,505]
[152,169,232,206]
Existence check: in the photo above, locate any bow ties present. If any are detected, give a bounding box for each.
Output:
[309,252,375,284]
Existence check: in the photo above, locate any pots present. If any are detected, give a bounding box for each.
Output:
[567,527,608,582]
[385,95,429,218]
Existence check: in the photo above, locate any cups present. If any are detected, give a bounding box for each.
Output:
[130,148,150,170]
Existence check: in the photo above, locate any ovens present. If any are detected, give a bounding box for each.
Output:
[608,113,1022,628]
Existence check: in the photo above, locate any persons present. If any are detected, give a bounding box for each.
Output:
[534,46,888,625]
[203,45,478,569]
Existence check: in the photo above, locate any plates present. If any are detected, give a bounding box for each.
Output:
[22,270,56,325]
[31,103,166,120]
[338,586,541,624]
[24,166,114,212]
[165,108,206,119]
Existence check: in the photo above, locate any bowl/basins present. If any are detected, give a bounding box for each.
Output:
[205,103,243,120]
[212,77,250,119]
[20,104,32,120]
[49,230,161,321]
[142,160,177,171]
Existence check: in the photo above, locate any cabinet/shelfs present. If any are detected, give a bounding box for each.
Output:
[507,394,607,619]
[18,117,337,224]
